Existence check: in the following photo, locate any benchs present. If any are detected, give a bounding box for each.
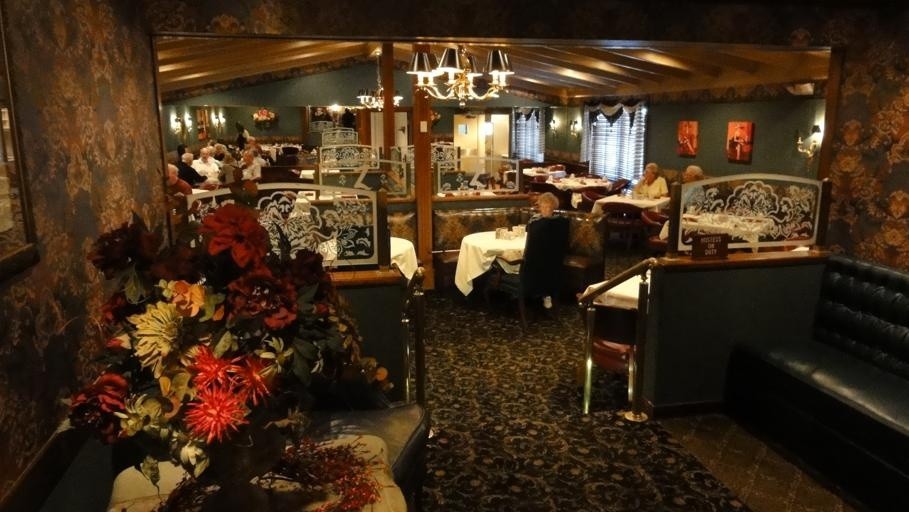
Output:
[764,254,909,511]
[433,206,604,294]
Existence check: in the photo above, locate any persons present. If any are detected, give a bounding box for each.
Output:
[682,164,706,185]
[523,191,561,231]
[164,134,298,240]
[308,106,358,131]
[627,161,670,255]
[232,120,252,149]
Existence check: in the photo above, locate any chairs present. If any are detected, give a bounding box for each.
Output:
[531,172,670,256]
[483,215,570,333]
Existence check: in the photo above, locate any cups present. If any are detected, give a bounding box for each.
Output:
[495,223,527,240]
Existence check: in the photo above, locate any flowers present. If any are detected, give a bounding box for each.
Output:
[58,196,396,493]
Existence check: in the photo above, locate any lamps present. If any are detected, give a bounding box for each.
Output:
[404,43,518,108]
[356,50,403,113]
